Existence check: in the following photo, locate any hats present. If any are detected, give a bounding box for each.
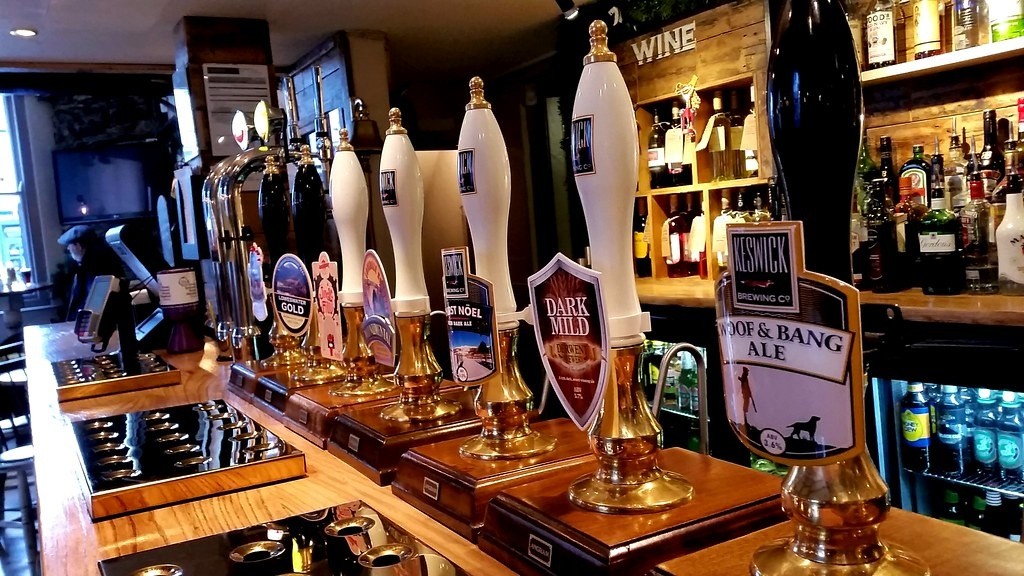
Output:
[57,225,94,245]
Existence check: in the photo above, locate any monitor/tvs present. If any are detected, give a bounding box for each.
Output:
[105,225,172,297]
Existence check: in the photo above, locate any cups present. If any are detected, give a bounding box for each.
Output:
[20,268,32,289]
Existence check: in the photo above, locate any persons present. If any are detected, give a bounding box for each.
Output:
[57,225,124,321]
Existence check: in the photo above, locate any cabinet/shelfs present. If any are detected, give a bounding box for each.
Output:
[615,0,1024,538]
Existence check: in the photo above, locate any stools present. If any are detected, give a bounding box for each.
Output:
[0,342,38,565]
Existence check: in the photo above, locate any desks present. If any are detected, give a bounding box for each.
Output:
[0,282,53,334]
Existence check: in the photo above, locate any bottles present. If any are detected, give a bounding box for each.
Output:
[900,380,1024,544]
[633,81,787,282]
[638,339,699,450]
[851,1,1024,298]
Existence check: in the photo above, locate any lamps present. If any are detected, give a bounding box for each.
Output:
[555,0,579,19]
